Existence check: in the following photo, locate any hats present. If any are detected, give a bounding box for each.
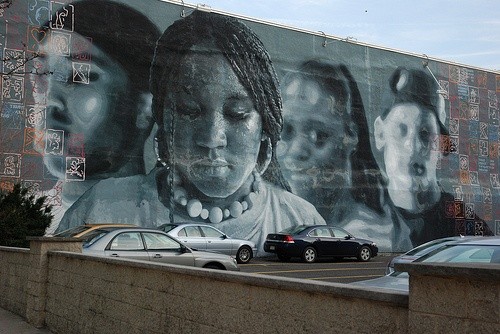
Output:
[381,67,458,155]
[37,0,164,86]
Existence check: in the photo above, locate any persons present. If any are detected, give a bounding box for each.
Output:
[274,60,412,255]
[373,68,495,248]
[32,1,164,237]
[53,14,326,253]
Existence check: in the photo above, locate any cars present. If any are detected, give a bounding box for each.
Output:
[155,222,259,264]
[264,224,379,263]
[53,223,139,238]
[80,227,241,271]
[346,235,500,291]
[383,236,497,276]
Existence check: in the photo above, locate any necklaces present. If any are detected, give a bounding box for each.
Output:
[166,171,264,223]
